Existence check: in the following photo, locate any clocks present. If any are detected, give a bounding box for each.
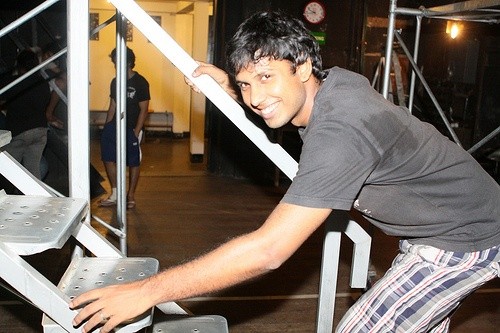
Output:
[303,1,325,24]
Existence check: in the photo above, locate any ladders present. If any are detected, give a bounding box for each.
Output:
[370,47,406,109]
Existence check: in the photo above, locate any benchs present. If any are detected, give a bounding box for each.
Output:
[90,110,173,138]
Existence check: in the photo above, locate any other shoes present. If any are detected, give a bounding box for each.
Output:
[50,119,64,129]
[100,199,117,206]
[127,201,135,208]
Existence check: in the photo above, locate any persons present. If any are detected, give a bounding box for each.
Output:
[69,8,500,333]
[95,46,151,210]
[1,29,68,196]
